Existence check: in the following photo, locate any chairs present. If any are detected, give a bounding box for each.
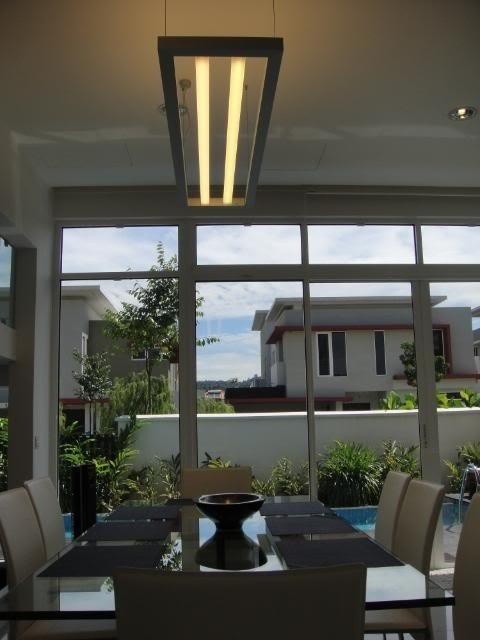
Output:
[450,489,480,639]
[21,475,72,562]
[179,466,255,499]
[373,470,412,558]
[102,560,371,640]
[0,482,120,640]
[362,478,448,639]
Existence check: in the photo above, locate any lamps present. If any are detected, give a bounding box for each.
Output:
[152,0,288,215]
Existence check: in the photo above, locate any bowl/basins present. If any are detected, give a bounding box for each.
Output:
[194,530,268,570]
[194,493,265,529]
